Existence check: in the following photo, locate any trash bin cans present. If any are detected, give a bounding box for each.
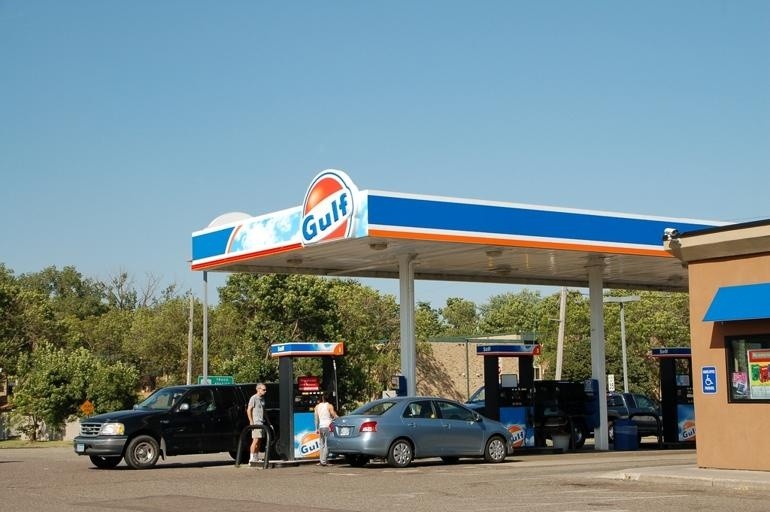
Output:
[614,419,638,451]
[551,432,571,452]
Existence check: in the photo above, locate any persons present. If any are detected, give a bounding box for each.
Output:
[247,382,267,466]
[315,391,339,466]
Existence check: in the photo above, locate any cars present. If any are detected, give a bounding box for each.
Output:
[327,396,514,468]
[441,380,663,449]
[74,383,309,470]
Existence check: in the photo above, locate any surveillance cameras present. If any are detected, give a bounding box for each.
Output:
[663,227,679,238]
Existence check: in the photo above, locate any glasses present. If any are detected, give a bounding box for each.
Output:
[260,388,266,391]
